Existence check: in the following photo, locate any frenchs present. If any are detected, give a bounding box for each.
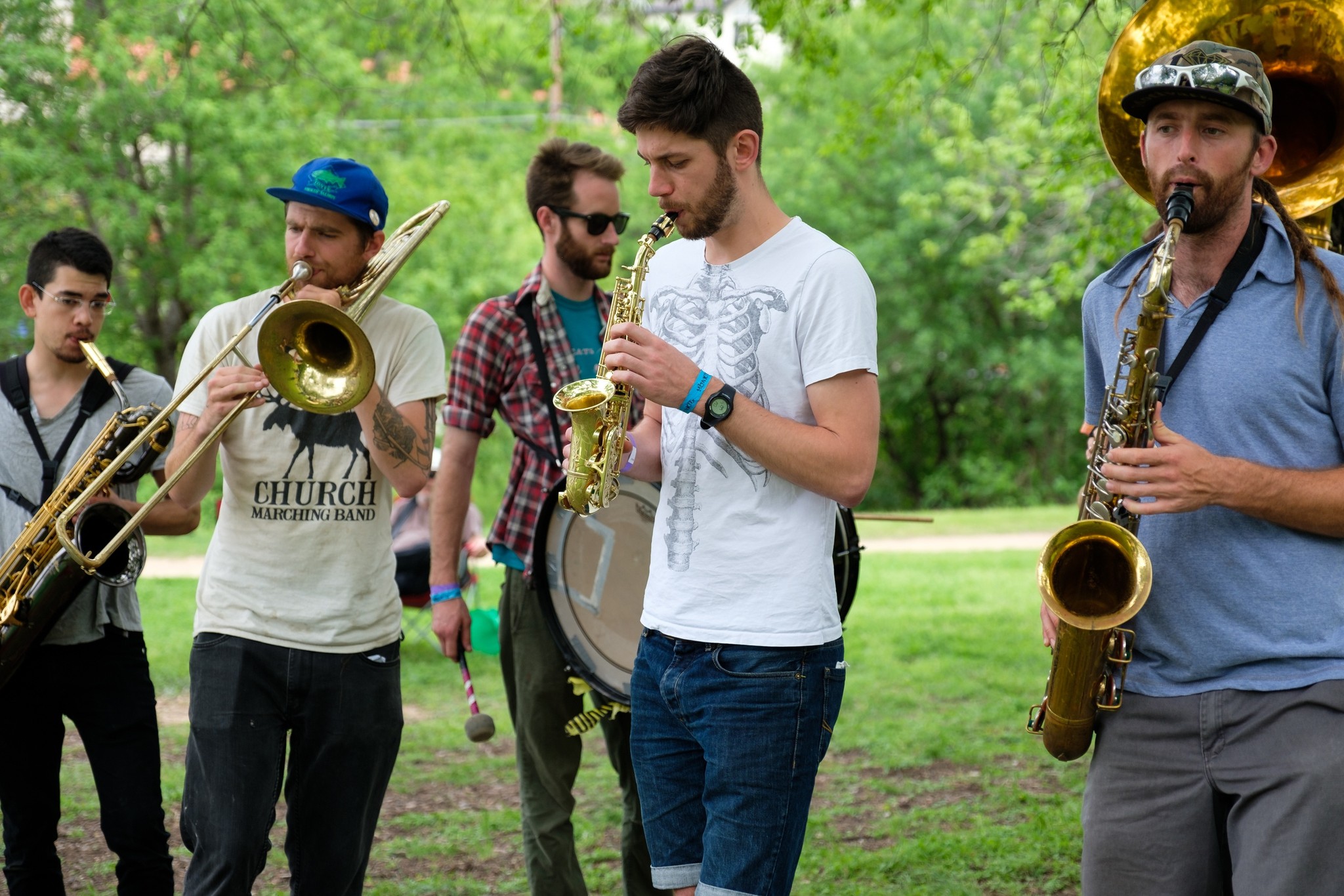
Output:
[1096,1,1344,252]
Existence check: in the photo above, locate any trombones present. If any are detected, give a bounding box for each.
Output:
[54,198,451,566]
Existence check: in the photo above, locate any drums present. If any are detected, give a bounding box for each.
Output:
[532,468,862,708]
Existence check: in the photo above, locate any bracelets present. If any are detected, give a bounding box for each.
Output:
[618,431,637,473]
[429,583,462,605]
[678,370,713,414]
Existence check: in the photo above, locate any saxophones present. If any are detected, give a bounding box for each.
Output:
[1027,180,1198,761]
[553,211,685,516]
[0,338,175,685]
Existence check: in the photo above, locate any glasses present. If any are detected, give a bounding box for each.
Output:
[550,209,631,235]
[1134,62,1271,116]
[31,281,117,316]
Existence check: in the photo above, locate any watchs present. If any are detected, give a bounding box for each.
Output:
[700,383,736,431]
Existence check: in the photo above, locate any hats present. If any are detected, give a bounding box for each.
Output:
[1122,41,1272,137]
[266,157,388,234]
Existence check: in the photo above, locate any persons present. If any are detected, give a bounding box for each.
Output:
[0,227,201,896]
[390,446,488,609]
[560,34,880,896]
[427,137,662,896]
[165,157,448,896]
[1040,40,1344,896]
